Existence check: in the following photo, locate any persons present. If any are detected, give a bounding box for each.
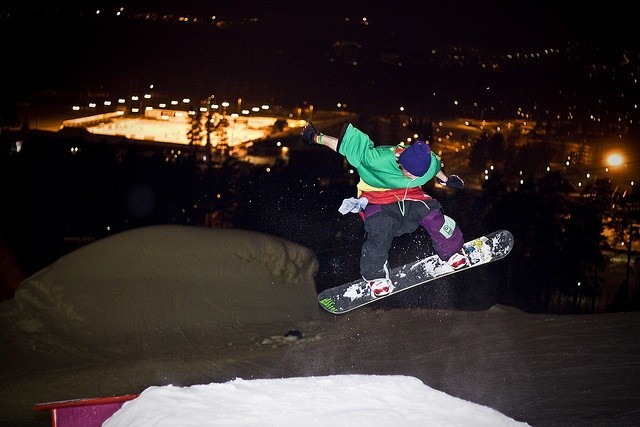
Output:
[300,118,467,298]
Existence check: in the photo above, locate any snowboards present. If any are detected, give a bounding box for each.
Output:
[317,230,515,314]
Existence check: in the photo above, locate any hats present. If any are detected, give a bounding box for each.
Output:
[399,141,431,177]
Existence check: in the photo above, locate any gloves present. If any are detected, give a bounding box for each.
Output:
[446,174,464,190]
[299,118,319,146]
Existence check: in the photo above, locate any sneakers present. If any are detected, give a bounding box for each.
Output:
[370,278,390,295]
[448,250,466,269]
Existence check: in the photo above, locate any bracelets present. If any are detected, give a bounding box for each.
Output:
[317,132,324,145]
